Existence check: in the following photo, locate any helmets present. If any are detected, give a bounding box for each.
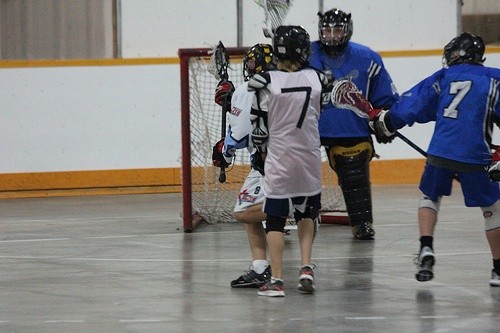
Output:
[273,25,311,67]
[318,9,353,51]
[442,32,485,67]
[243,44,276,82]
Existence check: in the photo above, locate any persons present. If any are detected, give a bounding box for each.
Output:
[308,8,399,239]
[373,31,500,286]
[249,24,328,297]
[213,43,278,287]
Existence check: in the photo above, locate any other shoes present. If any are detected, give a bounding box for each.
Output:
[354,222,375,239]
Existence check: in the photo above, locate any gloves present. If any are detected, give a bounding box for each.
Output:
[215,79,235,112]
[212,139,233,169]
[487,144,500,183]
[373,110,398,144]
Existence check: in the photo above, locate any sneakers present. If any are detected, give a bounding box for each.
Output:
[231,265,272,288]
[297,263,317,293]
[414,246,436,282]
[489,269,500,286]
[258,278,285,297]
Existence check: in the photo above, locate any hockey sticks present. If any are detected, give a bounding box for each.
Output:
[330,80,428,157]
[206,40,230,183]
[253,0,294,40]
